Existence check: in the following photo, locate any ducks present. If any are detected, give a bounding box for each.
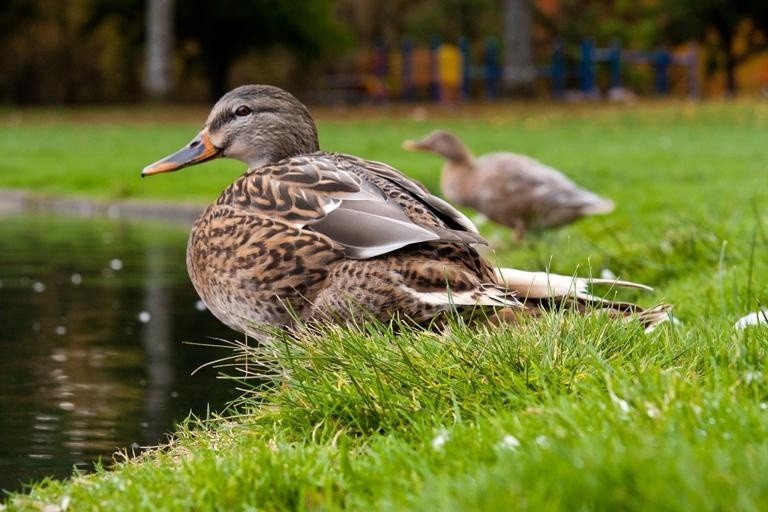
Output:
[401,129,615,243]
[140,84,675,358]
